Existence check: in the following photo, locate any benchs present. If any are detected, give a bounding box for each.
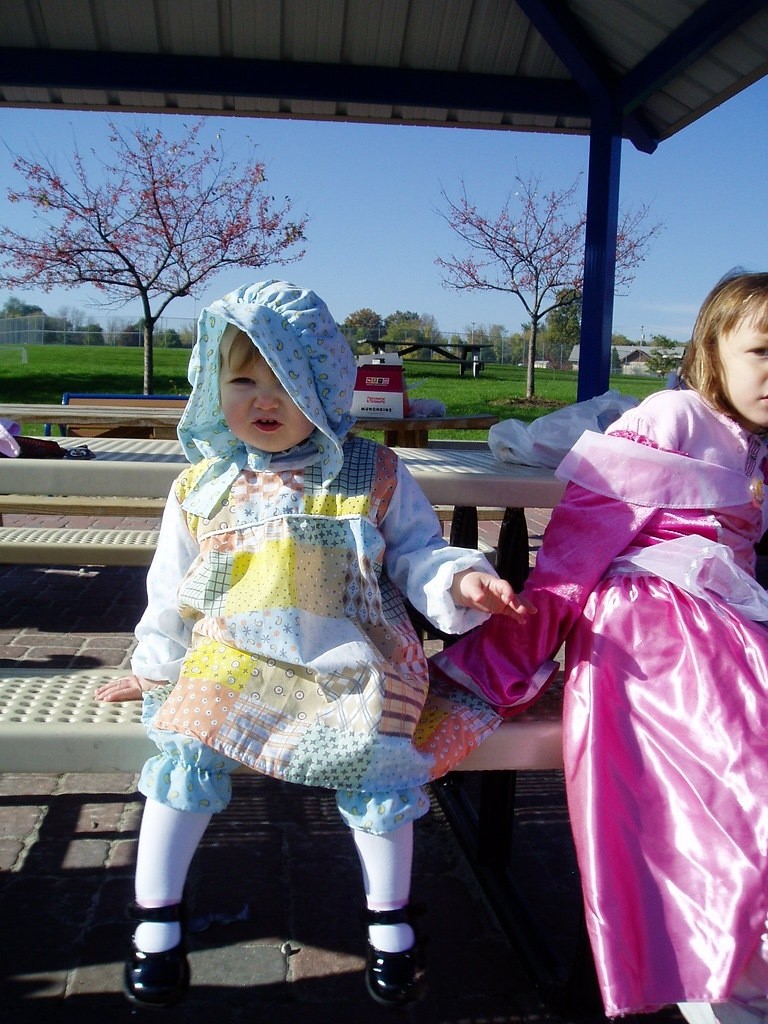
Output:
[0,393,564,1011]
[402,357,498,377]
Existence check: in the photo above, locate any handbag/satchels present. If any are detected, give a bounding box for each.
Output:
[488,389,639,470]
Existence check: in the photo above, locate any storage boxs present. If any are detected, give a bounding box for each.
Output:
[350,352,428,418]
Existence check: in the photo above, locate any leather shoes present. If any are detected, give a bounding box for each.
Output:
[363,907,432,1003]
[124,900,193,1009]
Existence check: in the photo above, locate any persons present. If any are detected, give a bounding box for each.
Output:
[429,271,768,1016]
[97,279,537,1005]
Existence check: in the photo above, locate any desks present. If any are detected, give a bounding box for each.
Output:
[351,415,499,448]
[356,338,494,377]
[1,437,562,593]
[0,403,185,438]
